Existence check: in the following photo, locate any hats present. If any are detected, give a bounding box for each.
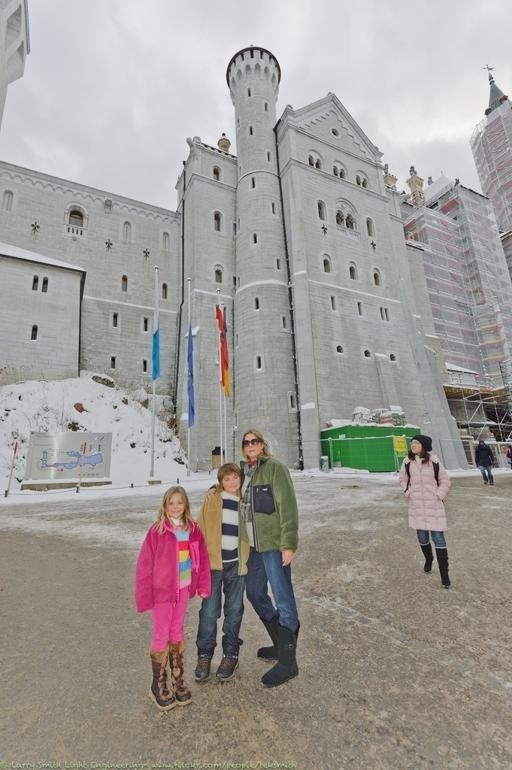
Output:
[411,435,432,451]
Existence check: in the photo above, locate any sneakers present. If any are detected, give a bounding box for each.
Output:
[194,649,214,682]
[215,650,240,681]
[490,481,494,485]
[484,480,488,484]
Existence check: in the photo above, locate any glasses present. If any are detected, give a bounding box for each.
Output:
[242,439,263,447]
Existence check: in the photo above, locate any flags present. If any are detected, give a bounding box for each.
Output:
[214,292,233,402]
[183,276,198,432]
[149,261,165,388]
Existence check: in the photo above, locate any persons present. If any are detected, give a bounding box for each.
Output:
[474,439,497,490]
[204,428,301,690]
[193,463,252,683]
[134,485,213,713]
[396,432,452,593]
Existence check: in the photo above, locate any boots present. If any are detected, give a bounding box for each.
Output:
[262,621,300,688]
[150,650,175,710]
[436,547,450,589]
[168,638,193,707]
[420,542,433,572]
[258,612,279,660]
